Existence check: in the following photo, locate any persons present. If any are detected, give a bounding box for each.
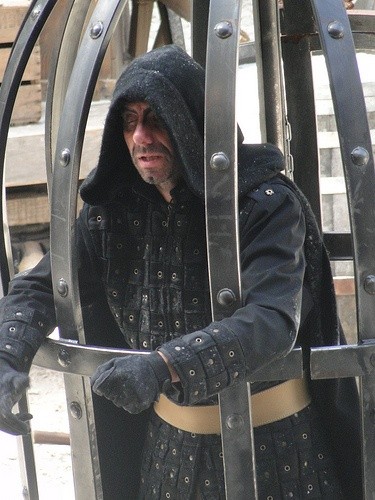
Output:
[0,44,364,500]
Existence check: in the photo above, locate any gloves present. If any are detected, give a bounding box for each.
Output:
[0,358,32,435]
[90,351,172,415]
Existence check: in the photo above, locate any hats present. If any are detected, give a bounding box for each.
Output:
[79,45,285,204]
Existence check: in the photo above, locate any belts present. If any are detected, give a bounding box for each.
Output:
[153,378,311,434]
